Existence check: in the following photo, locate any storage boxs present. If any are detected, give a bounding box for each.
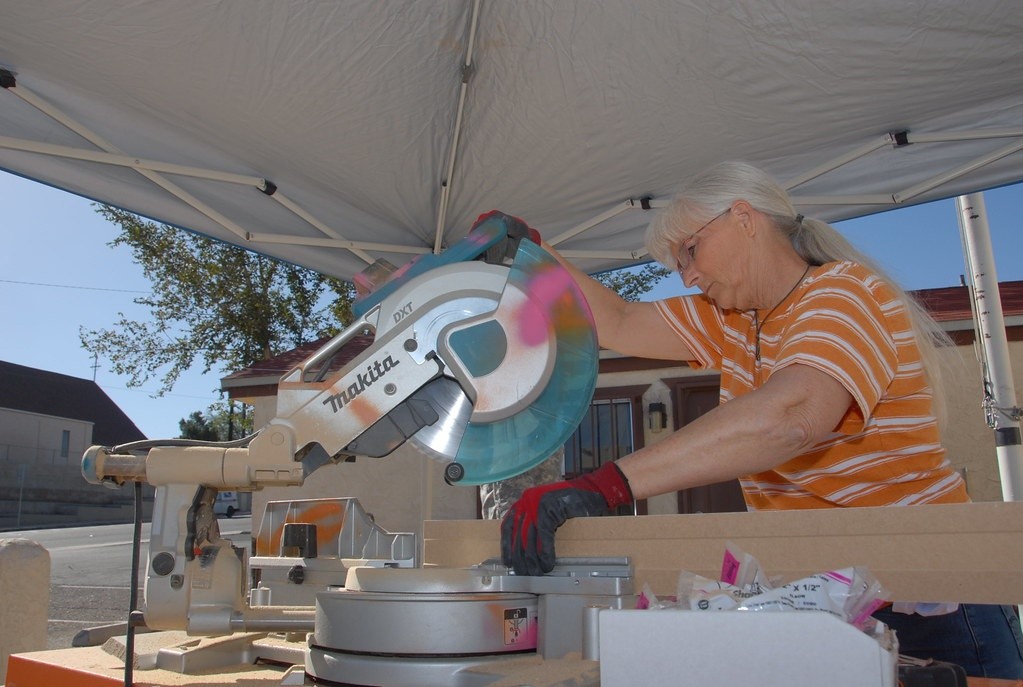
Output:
[599,608,899,687]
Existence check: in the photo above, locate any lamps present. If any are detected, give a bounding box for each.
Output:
[648,402,666,433]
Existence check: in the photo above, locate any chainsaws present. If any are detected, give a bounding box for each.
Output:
[79,211,636,687]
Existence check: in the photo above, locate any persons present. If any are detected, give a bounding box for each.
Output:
[468,161,1023,681]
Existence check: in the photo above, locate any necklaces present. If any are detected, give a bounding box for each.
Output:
[754,265,809,361]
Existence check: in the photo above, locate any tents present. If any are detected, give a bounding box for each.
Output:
[1,0,1022,280]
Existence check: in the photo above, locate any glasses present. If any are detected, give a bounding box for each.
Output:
[678,208,732,275]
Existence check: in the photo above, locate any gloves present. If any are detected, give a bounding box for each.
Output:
[470,210,541,263]
[500,461,633,576]
